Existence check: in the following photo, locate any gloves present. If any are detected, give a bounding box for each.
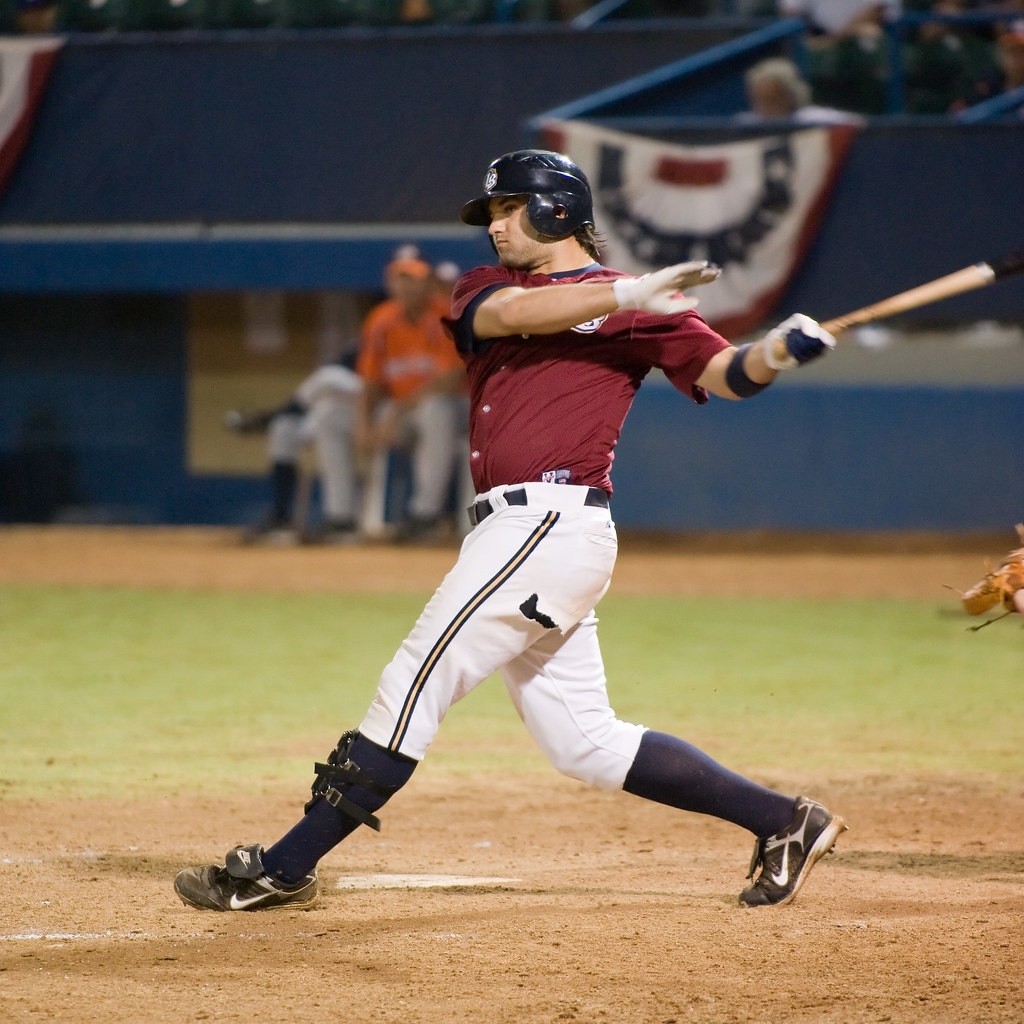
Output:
[612,260,722,316]
[763,313,836,370]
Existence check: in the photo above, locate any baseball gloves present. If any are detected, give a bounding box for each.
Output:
[959,544,1024,618]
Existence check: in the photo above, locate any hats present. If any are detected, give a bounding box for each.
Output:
[459,149,596,238]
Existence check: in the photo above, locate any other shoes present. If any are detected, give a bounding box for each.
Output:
[297,518,357,545]
[393,512,438,545]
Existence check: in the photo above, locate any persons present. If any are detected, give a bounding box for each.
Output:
[174,149,852,914]
[777,0,1024,112]
[0,0,59,36]
[234,351,365,545]
[357,242,471,543]
[743,56,812,116]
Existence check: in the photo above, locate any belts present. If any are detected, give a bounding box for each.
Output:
[467,489,608,525]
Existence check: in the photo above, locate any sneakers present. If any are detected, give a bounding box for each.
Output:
[738,796,850,909]
[173,844,320,912]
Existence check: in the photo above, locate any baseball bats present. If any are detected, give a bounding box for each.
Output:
[771,259,997,364]
